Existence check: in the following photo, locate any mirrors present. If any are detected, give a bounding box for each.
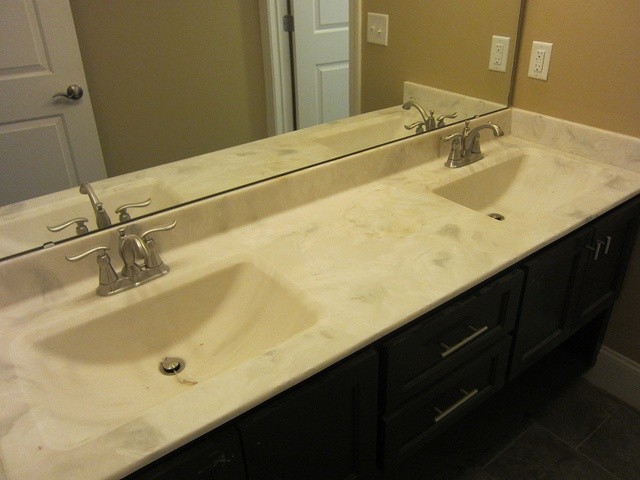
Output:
[0,0,528,264]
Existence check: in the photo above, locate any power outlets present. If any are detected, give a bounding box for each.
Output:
[528,40,553,81]
[488,35,510,72]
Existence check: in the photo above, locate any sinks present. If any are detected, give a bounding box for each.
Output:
[313,117,421,155]
[11,255,333,453]
[0,177,182,260]
[431,153,617,231]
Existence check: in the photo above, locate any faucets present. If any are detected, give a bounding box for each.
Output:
[401,101,436,130]
[116,228,151,276]
[463,119,504,155]
[79,182,111,228]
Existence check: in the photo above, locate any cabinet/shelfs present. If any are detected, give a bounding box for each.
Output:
[382,266,525,480]
[126,366,355,480]
[505,200,640,434]
